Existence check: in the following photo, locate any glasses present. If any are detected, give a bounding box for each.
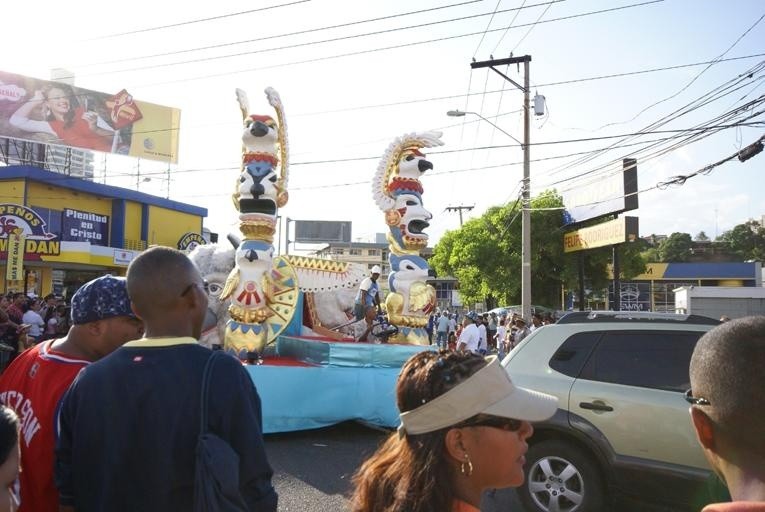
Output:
[182,277,211,296]
[683,388,711,407]
[457,417,523,432]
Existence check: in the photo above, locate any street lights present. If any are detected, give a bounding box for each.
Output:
[448,110,534,326]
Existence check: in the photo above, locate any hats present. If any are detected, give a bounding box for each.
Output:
[464,311,525,323]
[371,265,382,275]
[397,353,559,439]
[69,273,138,325]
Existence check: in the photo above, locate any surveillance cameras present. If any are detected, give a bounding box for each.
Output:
[534,95,546,115]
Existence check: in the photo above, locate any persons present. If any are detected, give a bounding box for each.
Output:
[47,243,280,511]
[350,306,384,345]
[354,265,384,319]
[679,313,765,512]
[1,273,147,512]
[7,84,129,157]
[0,290,71,364]
[343,349,563,512]
[0,401,27,512]
[422,306,556,364]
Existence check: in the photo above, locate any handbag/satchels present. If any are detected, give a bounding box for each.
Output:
[191,431,251,512]
